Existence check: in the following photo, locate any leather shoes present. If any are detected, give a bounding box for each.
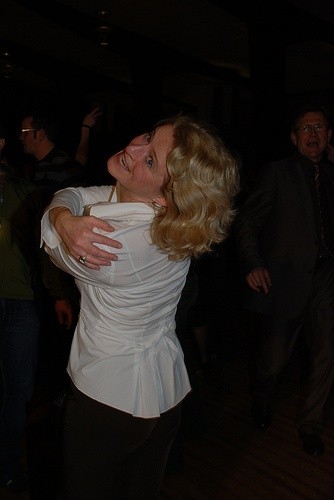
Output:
[298,432,324,456]
[252,411,272,432]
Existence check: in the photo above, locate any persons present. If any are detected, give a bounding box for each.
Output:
[1,96,333,500]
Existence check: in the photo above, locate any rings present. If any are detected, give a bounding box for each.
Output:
[79,253,89,264]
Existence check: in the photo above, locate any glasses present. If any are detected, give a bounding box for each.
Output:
[19,128,36,136]
[297,124,328,132]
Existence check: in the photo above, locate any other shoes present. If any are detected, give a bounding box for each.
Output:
[204,365,225,387]
[6,475,30,496]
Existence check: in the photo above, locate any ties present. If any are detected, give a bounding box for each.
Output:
[314,164,325,258]
